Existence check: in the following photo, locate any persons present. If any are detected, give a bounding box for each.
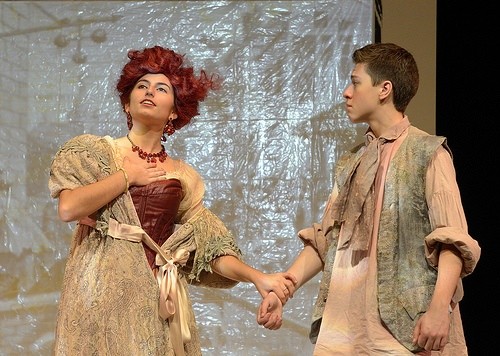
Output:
[48,44,297,356]
[255,43,482,356]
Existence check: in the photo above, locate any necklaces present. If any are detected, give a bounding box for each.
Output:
[127,133,168,162]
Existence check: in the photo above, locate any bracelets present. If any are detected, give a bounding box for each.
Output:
[117,167,129,193]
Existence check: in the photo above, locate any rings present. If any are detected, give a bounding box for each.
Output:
[282,286,287,291]
